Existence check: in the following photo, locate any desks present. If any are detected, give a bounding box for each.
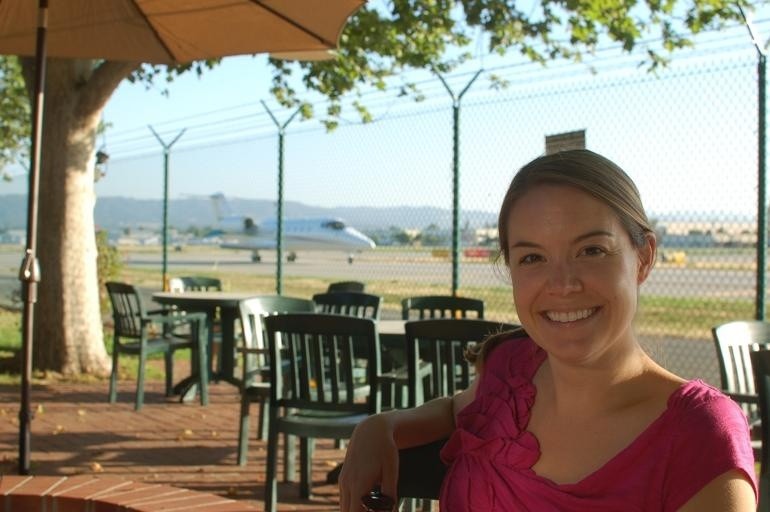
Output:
[148,288,259,399]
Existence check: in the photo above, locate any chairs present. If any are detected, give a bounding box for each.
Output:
[162,276,223,396]
[393,320,523,512]
[238,281,383,495]
[401,294,486,407]
[264,313,421,512]
[746,348,769,511]
[708,318,770,459]
[103,279,210,411]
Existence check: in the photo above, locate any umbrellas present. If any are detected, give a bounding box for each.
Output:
[0,1,372,475]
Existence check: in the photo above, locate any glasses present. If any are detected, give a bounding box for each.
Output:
[360,490,395,512]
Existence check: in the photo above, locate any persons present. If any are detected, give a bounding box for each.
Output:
[336,150,760,512]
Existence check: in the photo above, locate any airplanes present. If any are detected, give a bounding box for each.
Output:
[210,190,377,265]
